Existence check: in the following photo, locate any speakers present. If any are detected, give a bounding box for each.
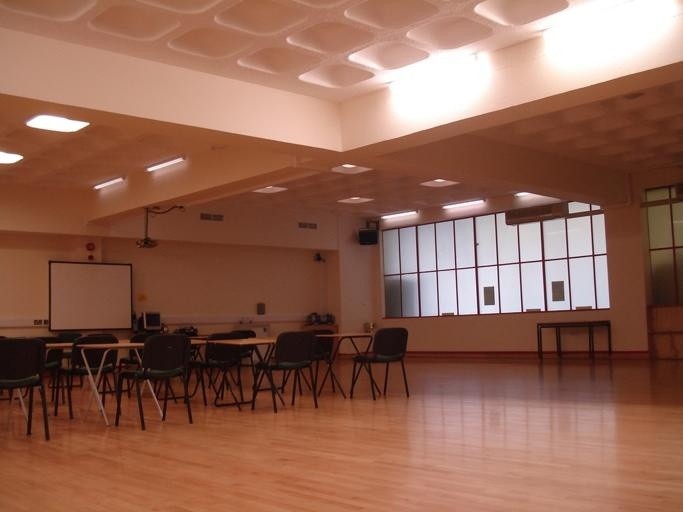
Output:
[257,304,264,314]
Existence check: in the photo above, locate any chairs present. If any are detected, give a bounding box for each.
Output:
[115,332,195,431]
[350,328,409,401]
[1,336,50,441]
[33,332,285,418]
[254,330,319,413]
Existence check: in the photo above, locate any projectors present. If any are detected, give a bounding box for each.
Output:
[136,239,158,249]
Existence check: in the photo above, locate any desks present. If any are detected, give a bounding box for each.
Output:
[315,332,382,399]
[536,321,612,360]
[76,340,208,425]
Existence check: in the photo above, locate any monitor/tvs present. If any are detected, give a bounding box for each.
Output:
[138,311,162,331]
[359,229,377,245]
